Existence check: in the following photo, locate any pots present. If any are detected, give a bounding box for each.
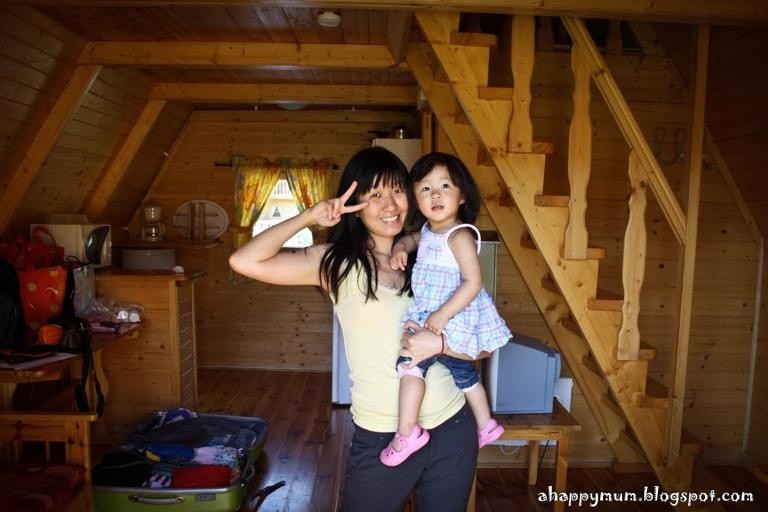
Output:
[367,124,408,139]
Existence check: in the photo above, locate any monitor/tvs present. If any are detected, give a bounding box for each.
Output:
[482,332,562,415]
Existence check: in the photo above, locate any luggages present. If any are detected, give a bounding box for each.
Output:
[89,413,268,511]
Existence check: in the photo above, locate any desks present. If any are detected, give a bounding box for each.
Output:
[1,323,141,412]
[465,397,582,512]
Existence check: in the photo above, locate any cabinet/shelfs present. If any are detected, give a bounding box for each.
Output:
[95,270,208,439]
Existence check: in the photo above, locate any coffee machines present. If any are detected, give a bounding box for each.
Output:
[143,202,163,241]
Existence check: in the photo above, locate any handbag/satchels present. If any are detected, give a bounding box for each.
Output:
[0,227,99,345]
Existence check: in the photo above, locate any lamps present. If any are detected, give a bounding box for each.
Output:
[141,204,166,249]
[318,9,342,29]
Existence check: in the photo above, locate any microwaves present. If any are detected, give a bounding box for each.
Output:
[29,223,113,269]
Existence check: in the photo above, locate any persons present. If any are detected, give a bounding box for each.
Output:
[229,145,512,511]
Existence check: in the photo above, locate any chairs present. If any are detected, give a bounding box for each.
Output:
[0,414,92,511]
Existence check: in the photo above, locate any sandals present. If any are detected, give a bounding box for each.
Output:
[378,423,430,467]
[477,418,504,449]
